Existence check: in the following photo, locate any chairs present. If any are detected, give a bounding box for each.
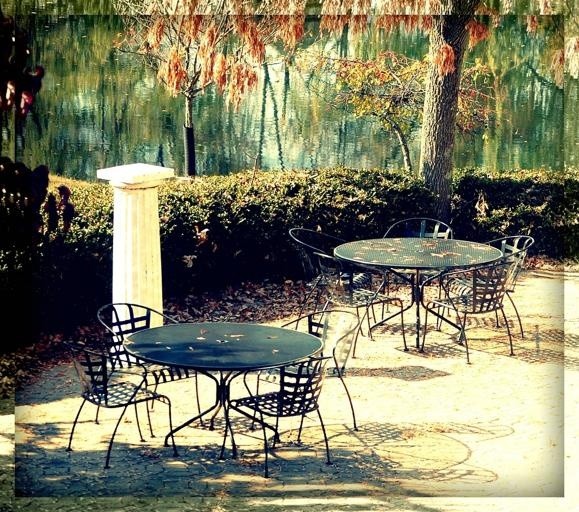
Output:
[96,302,206,428]
[249,309,359,433]
[62,341,179,469]
[219,355,334,476]
[288,217,534,365]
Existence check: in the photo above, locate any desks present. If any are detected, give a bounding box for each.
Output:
[123,323,326,460]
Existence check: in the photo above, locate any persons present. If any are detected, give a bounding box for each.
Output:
[5,64,45,138]
